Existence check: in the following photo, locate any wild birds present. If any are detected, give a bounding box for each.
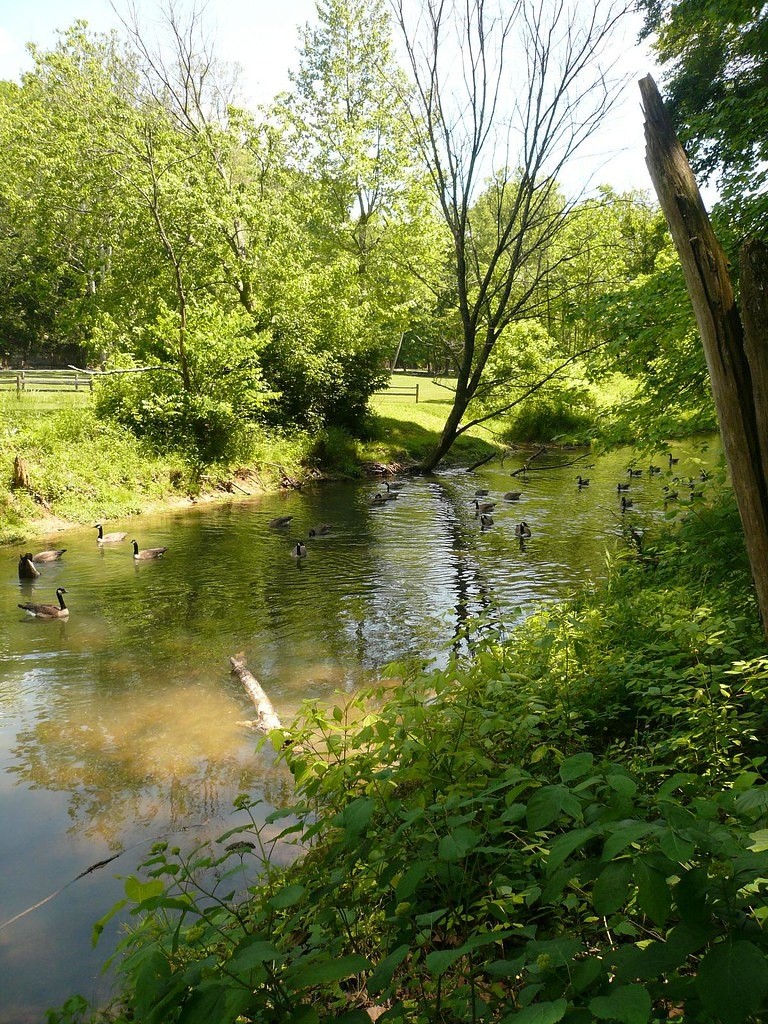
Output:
[14,451,709,622]
